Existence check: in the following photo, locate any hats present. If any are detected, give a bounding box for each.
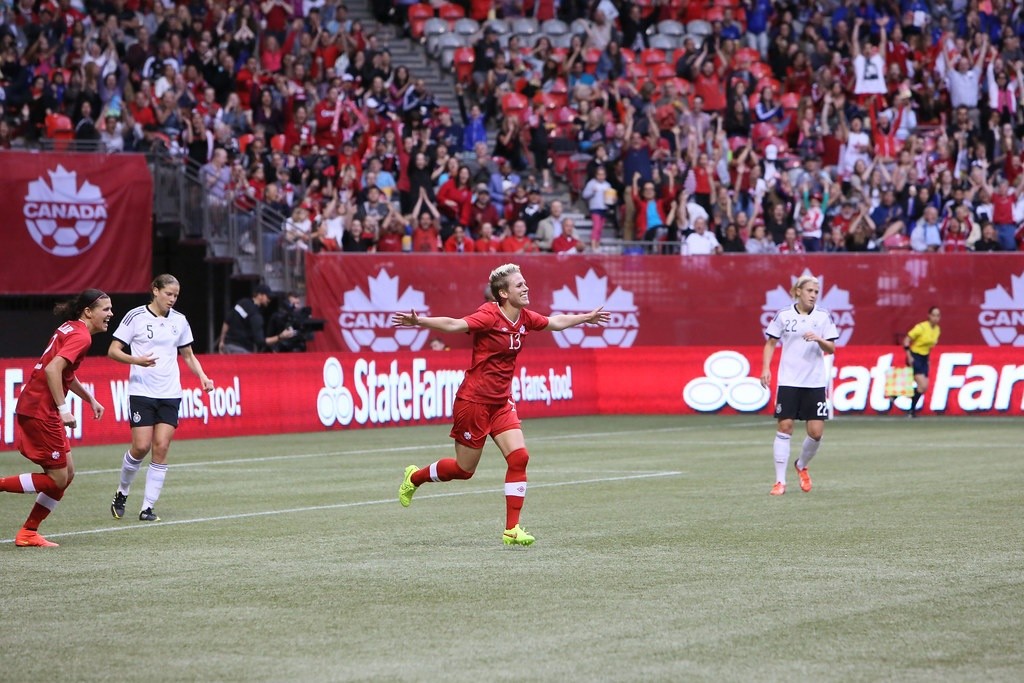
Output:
[254,285,277,298]
[288,289,304,297]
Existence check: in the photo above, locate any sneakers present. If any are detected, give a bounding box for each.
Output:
[111,491,128,520]
[502,524,535,547]
[795,459,812,492]
[16,528,59,547]
[399,465,420,507]
[770,482,786,496]
[139,507,161,522]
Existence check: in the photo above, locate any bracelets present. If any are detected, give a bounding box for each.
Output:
[904,346,909,350]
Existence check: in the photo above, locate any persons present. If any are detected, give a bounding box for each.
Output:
[0,288,113,547]
[107,274,214,522]
[760,275,840,495]
[391,263,612,548]
[0,0,1023,353]
[903,305,941,417]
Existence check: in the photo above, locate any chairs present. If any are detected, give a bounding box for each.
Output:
[410,0,803,200]
